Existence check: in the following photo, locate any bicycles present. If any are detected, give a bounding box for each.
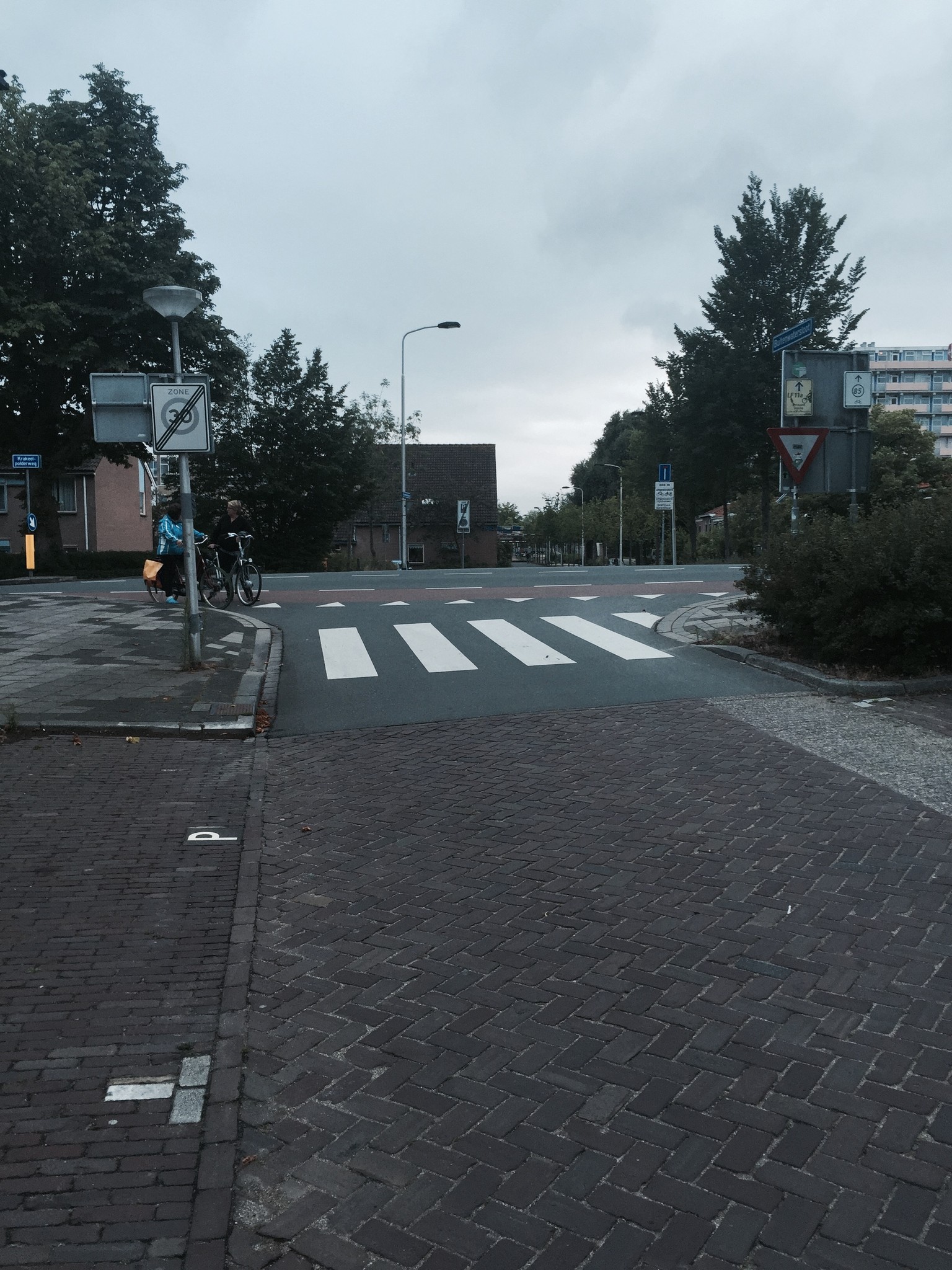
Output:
[146,529,262,610]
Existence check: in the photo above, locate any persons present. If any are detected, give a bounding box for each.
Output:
[156,504,205,604]
[526,553,529,561]
[208,500,261,604]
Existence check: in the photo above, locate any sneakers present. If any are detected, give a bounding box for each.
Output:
[248,596,260,601]
[166,597,179,604]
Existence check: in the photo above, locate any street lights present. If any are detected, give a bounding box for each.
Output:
[142,285,222,674]
[400,321,461,570]
[523,462,624,567]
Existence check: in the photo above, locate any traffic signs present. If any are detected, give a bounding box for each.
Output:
[785,378,814,417]
[844,370,871,409]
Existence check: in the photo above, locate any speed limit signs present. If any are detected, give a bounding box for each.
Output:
[149,384,212,452]
